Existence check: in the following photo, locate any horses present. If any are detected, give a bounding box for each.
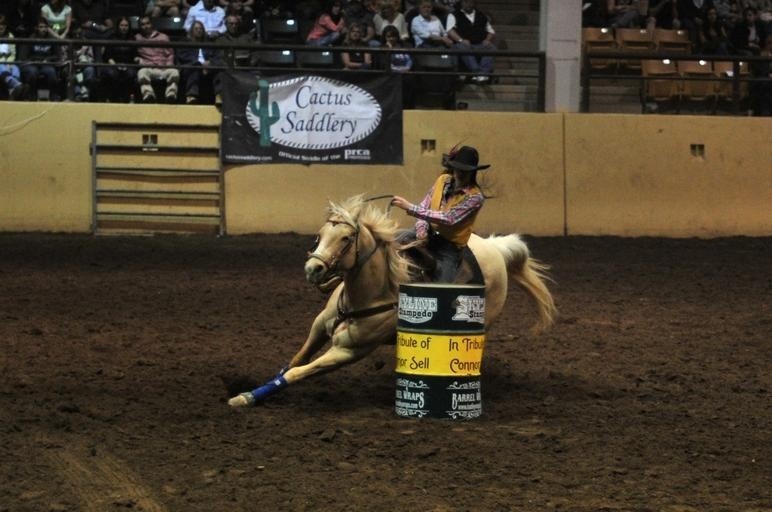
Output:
[227,191,563,409]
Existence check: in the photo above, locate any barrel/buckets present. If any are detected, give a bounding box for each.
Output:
[394,280,485,424]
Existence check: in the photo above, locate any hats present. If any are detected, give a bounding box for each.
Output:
[443,146,491,172]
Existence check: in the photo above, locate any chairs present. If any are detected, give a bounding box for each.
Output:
[582,27,751,113]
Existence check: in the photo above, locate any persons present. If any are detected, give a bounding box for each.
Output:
[316,143,491,295]
[305,0,495,82]
[0,0,260,105]
[581,0,770,67]
